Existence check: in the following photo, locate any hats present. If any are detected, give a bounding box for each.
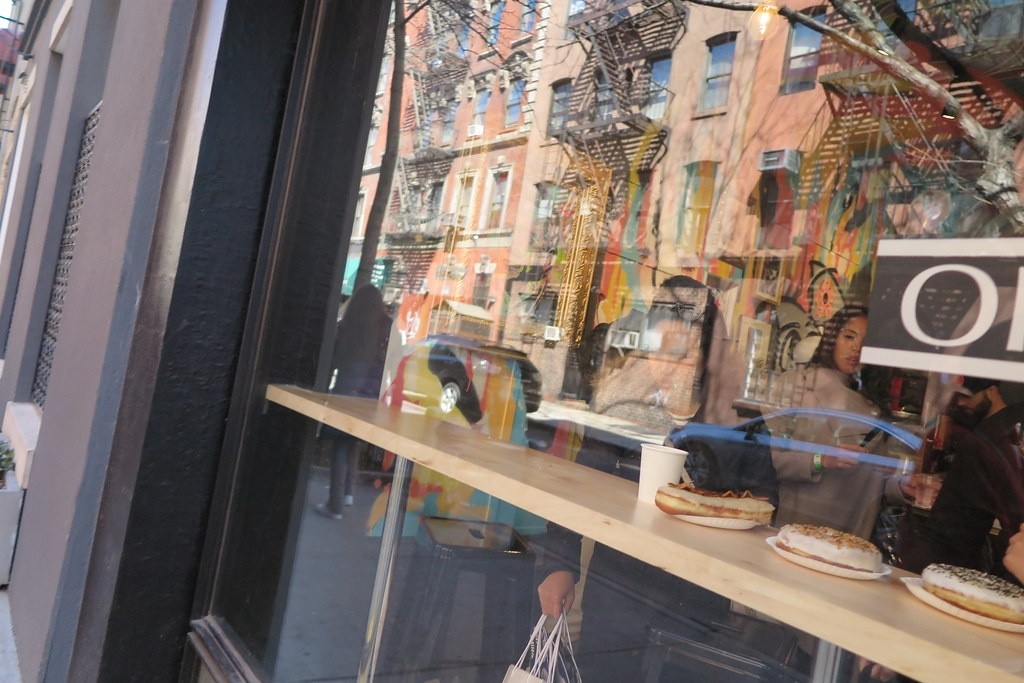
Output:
[953,375,1001,396]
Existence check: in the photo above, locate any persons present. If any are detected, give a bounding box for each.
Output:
[539,275,778,683]
[318,285,394,517]
[753,306,1024,683]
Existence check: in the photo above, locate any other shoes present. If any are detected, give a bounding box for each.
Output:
[344,494,353,505]
[316,503,342,519]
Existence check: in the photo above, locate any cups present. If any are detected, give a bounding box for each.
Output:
[637,443,689,505]
[913,473,943,510]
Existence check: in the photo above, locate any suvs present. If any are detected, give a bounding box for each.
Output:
[381,333,542,422]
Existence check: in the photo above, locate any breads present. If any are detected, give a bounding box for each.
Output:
[777,523,881,574]
[654,483,774,527]
[921,563,1024,626]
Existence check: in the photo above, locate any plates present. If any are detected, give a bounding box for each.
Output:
[766,536,893,580]
[671,514,761,530]
[900,577,1024,634]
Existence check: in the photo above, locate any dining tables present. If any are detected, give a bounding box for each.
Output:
[262,382,1024,683]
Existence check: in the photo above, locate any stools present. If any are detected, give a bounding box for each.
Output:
[631,598,802,683]
[379,511,536,683]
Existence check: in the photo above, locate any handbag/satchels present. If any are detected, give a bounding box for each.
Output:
[502,609,582,683]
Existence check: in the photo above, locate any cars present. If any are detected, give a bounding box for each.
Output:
[661,409,922,571]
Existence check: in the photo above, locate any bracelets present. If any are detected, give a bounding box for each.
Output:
[814,453,822,470]
